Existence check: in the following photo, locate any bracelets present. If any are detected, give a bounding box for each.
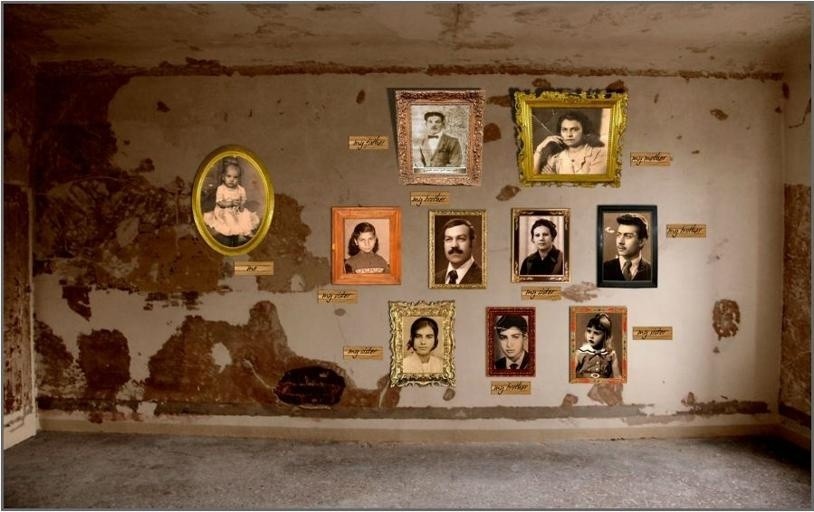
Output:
[537,149,543,154]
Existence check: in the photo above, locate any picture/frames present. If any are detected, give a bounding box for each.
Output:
[487,307,537,377]
[330,207,404,286]
[191,144,276,257]
[513,90,629,188]
[390,299,457,387]
[394,89,486,187]
[596,205,658,289]
[428,209,489,290]
[569,306,628,383]
[512,207,572,283]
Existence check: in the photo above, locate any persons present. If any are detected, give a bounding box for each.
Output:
[402,317,442,375]
[520,219,563,276]
[576,312,621,379]
[493,314,529,370]
[534,110,608,175]
[436,218,482,284]
[202,157,259,238]
[345,223,389,274]
[413,112,463,168]
[603,215,652,280]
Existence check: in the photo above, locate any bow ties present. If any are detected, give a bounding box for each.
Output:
[428,135,440,139]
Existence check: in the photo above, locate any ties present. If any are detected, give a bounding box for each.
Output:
[447,271,457,285]
[509,364,519,369]
[621,260,633,279]
